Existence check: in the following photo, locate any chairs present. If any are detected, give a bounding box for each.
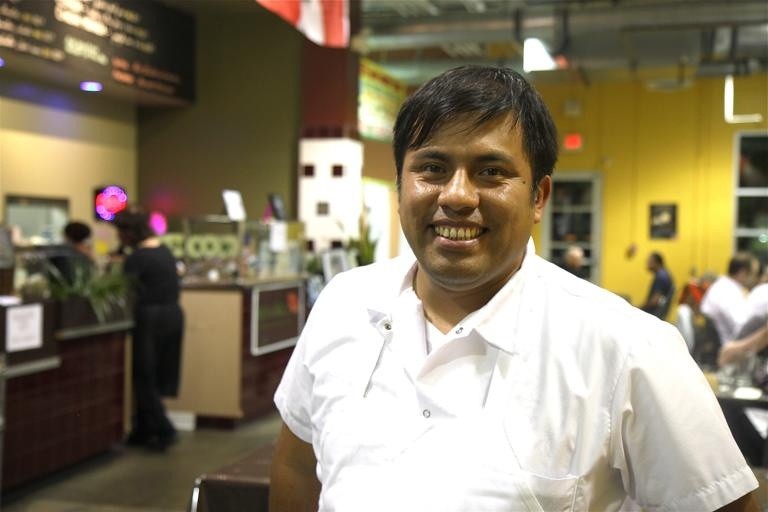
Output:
[654,284,720,374]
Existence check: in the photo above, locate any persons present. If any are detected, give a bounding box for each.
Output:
[717,282,768,402]
[64,221,96,260]
[637,253,675,321]
[698,252,758,367]
[267,66,761,511]
[114,212,184,457]
[554,246,583,277]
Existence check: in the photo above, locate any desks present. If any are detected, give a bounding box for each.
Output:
[704,373,767,470]
[189,444,274,512]
